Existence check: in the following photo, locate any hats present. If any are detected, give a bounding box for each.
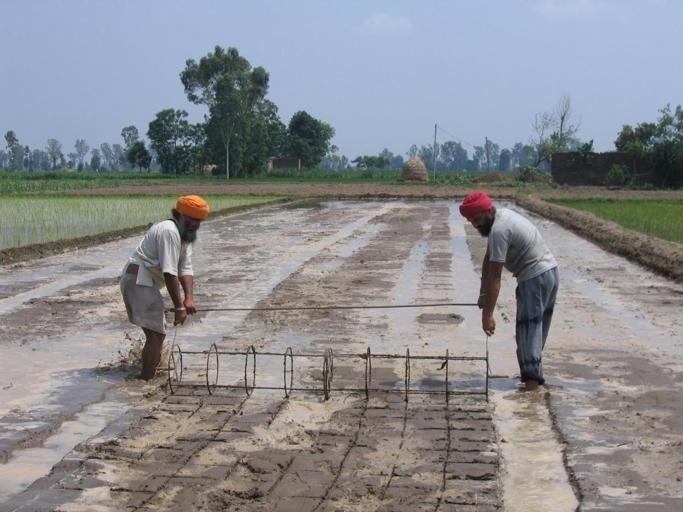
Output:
[459,192,493,218]
[176,194,208,220]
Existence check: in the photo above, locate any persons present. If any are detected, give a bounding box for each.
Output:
[119,194,209,380]
[459,192,560,401]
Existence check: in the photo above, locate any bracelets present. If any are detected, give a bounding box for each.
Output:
[174,306,186,312]
